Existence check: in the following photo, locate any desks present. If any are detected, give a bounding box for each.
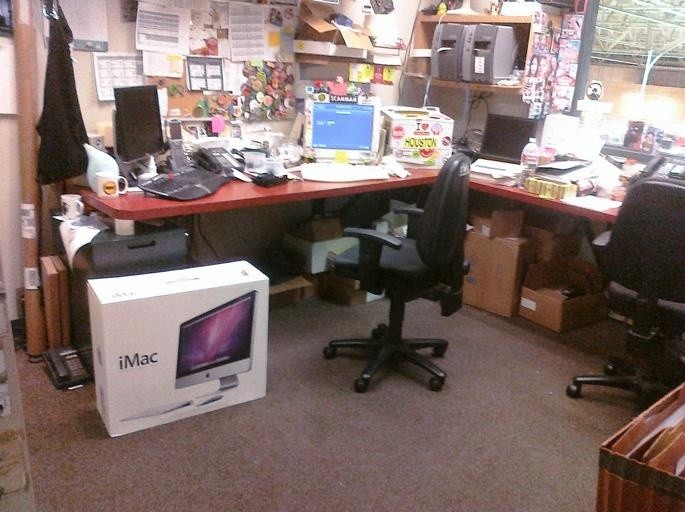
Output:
[74,154,623,221]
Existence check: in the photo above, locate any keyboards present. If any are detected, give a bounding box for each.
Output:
[119,400,191,422]
[299,162,390,184]
[137,170,227,202]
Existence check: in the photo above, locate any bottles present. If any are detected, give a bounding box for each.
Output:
[517,137,539,190]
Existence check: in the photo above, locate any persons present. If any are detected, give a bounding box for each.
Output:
[590,88,598,99]
[270,9,281,22]
[527,55,547,77]
[533,13,545,25]
[642,132,654,151]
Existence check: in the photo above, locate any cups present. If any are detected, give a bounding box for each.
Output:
[59,193,85,219]
[264,158,283,174]
[243,151,266,168]
[94,172,129,200]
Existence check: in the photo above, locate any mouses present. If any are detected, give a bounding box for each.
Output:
[194,394,221,408]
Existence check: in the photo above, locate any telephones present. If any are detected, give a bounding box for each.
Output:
[42,345,91,389]
[193,148,244,175]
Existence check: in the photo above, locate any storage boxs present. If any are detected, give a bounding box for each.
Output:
[297,2,374,50]
[380,106,455,165]
[337,289,385,307]
[88,221,188,280]
[523,226,564,261]
[86,260,271,438]
[284,232,361,275]
[518,261,608,333]
[470,209,525,239]
[462,238,531,318]
[305,217,343,242]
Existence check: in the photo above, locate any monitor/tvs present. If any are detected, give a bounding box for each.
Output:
[301,93,382,164]
[474,112,538,169]
[175,291,261,397]
[111,83,166,174]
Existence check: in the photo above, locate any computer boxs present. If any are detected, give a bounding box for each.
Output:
[430,24,467,83]
[460,24,526,84]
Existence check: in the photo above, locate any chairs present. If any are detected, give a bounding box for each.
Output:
[566,177,685,404]
[324,152,471,394]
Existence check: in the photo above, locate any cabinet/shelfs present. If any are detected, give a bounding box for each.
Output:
[404,0,563,96]
